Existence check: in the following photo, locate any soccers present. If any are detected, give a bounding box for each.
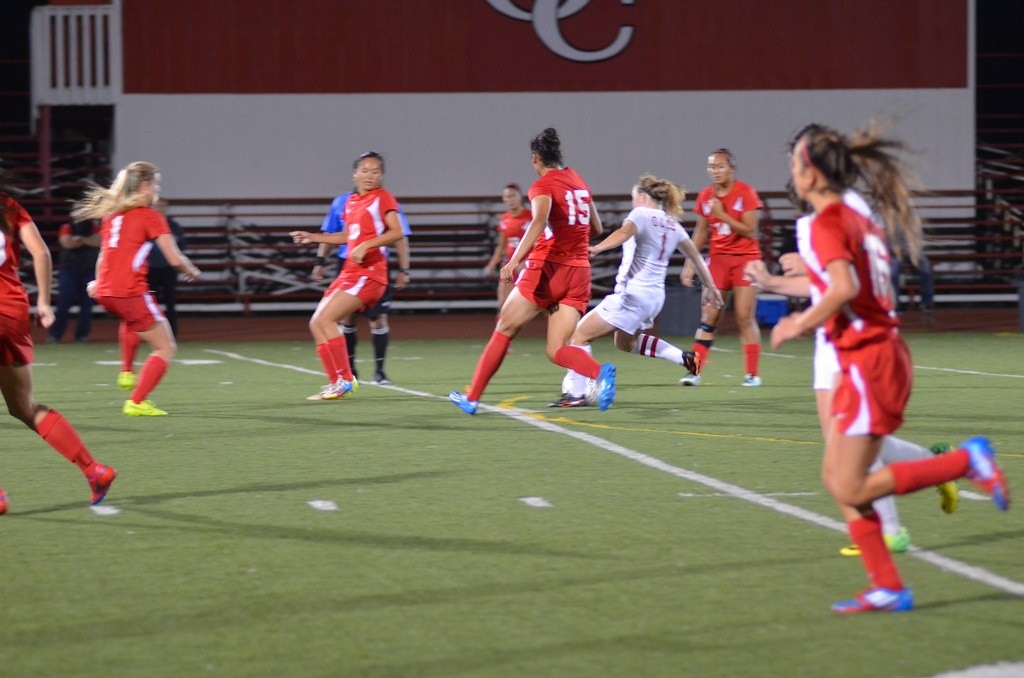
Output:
[562,371,597,396]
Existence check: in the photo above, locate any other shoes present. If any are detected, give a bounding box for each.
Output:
[45,335,58,344]
[74,336,89,344]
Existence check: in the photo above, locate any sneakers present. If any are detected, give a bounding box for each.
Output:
[117,371,138,391]
[679,373,702,387]
[592,362,618,409]
[961,435,1010,512]
[930,442,960,514]
[449,391,478,414]
[307,383,332,400]
[124,399,168,416]
[830,581,912,616]
[371,371,391,385]
[0,490,9,514]
[838,526,910,558]
[742,372,762,386]
[321,375,359,401]
[546,393,588,407]
[681,351,703,376]
[87,463,117,504]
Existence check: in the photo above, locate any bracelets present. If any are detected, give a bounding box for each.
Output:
[397,268,410,276]
[314,256,324,266]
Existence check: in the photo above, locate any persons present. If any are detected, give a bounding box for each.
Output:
[743,122,1009,615]
[548,173,723,409]
[43,198,185,343]
[0,187,118,515]
[92,162,202,418]
[682,148,764,385]
[450,126,617,419]
[289,153,412,410]
[487,182,532,319]
